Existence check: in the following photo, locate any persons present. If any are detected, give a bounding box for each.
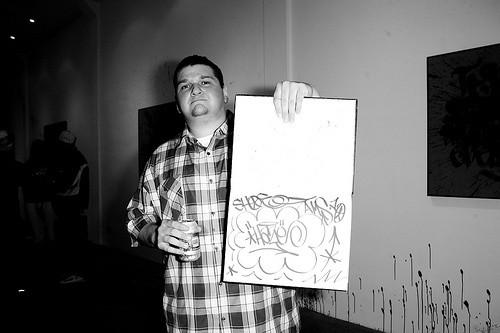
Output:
[22,139,55,244]
[0,129,29,293]
[52,130,90,284]
[124,54,320,333]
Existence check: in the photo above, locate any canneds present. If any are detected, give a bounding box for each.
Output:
[178,219,201,261]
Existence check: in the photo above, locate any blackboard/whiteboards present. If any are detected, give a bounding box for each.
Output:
[221,94,358,292]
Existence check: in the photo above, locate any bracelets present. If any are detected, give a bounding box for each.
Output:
[296,81,313,97]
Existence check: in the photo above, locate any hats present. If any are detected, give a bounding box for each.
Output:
[58,131,78,144]
